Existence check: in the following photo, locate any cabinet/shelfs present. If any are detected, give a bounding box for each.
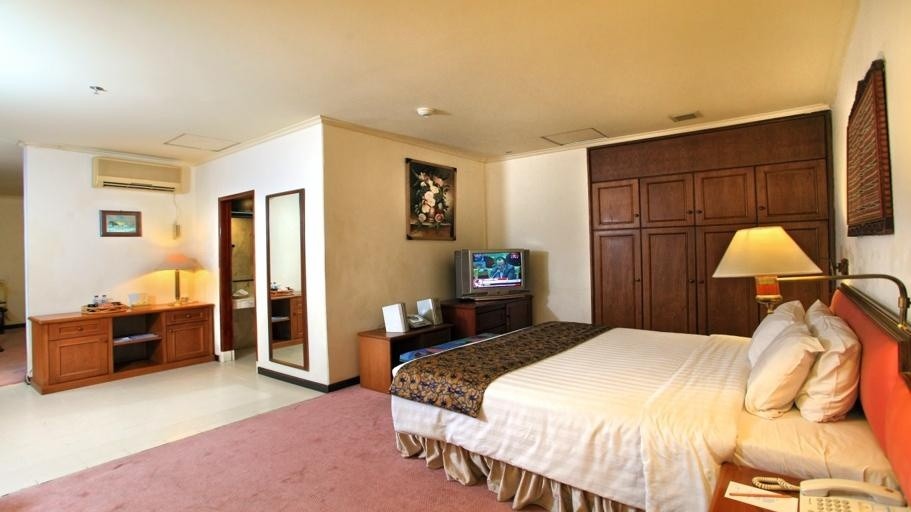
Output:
[589,173,642,330]
[358,322,457,392]
[639,167,760,337]
[111,309,166,380]
[28,315,112,394]
[270,291,304,349]
[440,295,534,335]
[754,158,830,309]
[146,306,214,364]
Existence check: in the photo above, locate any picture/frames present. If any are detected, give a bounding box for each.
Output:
[405,156,457,240]
[101,211,142,237]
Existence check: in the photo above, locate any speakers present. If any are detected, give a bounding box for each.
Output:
[381,302,409,333]
[416,297,443,325]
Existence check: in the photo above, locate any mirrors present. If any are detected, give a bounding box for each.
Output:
[265,188,308,371]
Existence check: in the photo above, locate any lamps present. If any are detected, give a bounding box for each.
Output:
[711,227,911,325]
[155,254,203,303]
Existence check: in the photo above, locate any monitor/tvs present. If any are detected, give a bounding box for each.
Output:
[454,249,528,301]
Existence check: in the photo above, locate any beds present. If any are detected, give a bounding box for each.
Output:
[390,280,911,512]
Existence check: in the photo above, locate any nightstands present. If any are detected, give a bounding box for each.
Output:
[707,463,800,512]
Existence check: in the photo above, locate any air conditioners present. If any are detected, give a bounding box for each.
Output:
[93,157,190,194]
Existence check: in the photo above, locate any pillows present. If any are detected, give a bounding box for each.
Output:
[793,316,862,423]
[747,299,803,363]
[745,324,823,417]
[806,299,835,325]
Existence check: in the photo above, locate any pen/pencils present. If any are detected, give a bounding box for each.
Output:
[729,493,792,498]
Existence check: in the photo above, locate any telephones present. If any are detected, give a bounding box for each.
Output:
[799,478,911,512]
[407,313,433,328]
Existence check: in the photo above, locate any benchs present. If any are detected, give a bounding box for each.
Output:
[400,332,500,361]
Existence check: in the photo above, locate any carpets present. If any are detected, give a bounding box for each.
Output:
[0,384,542,512]
[0,328,27,386]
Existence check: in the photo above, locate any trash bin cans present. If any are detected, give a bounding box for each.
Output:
[0,302,8,334]
